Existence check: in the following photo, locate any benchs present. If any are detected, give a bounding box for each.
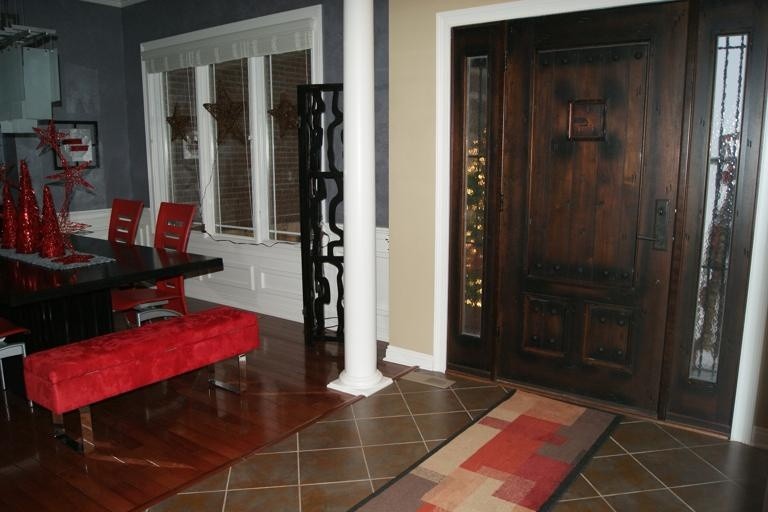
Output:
[24,305,261,456]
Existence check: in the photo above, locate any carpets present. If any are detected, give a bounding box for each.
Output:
[346,389,626,512]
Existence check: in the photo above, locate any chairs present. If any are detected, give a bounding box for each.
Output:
[1,316,33,421]
[108,198,197,328]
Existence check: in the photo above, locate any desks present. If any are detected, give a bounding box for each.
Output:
[1,233,223,417]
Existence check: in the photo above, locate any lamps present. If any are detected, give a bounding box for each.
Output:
[1,1,61,135]
